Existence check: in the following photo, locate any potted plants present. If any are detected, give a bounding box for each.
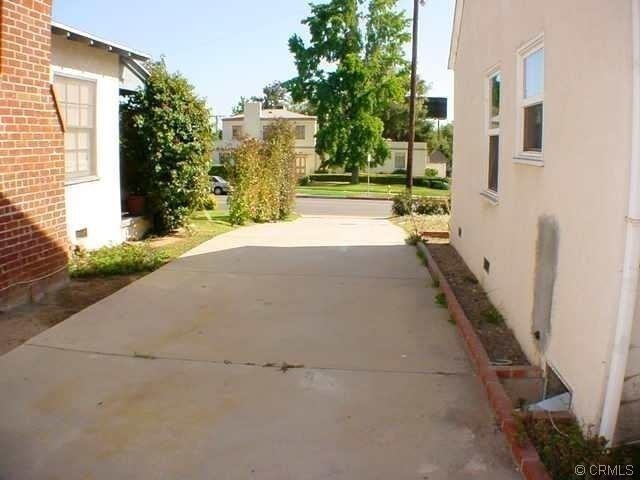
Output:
[127,186,145,215]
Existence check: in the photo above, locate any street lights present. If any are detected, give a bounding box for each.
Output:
[405,0,427,198]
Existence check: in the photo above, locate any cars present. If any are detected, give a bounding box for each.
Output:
[207,174,230,194]
